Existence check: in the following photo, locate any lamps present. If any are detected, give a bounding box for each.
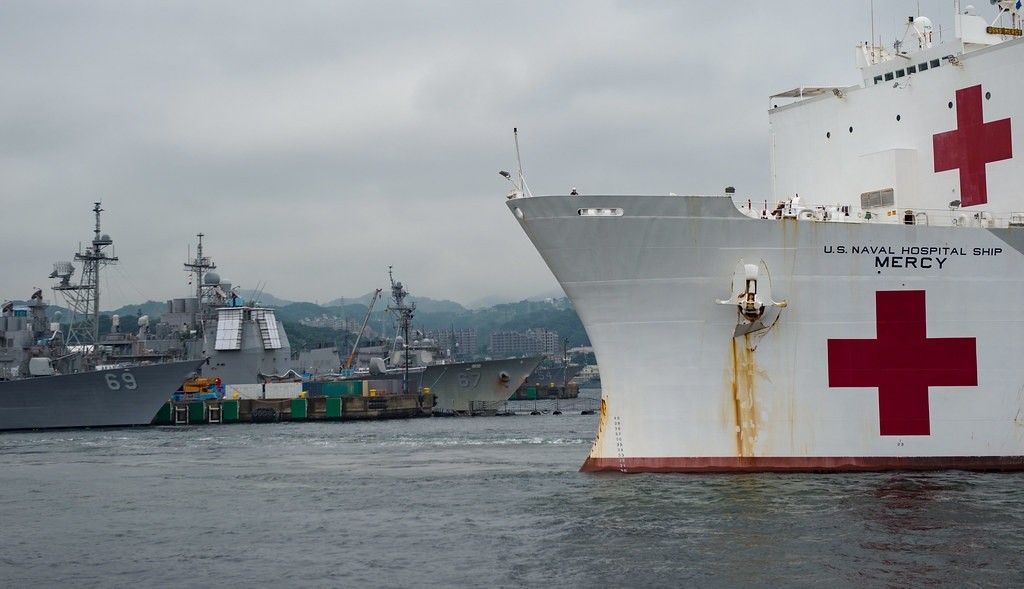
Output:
[893,76,912,92]
[947,54,964,68]
[832,88,843,99]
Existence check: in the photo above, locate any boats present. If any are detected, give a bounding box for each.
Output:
[96,231,547,418]
[500,1,1024,474]
[1,199,210,431]
